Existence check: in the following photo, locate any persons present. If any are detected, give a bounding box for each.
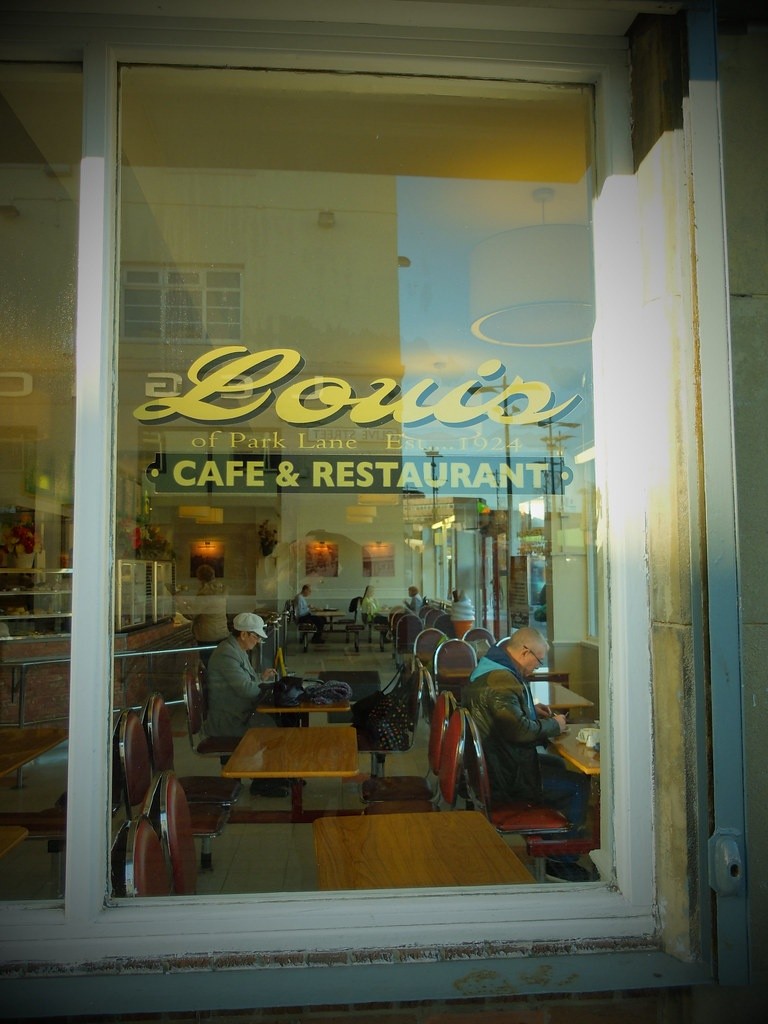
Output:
[362,585,392,643]
[293,584,326,643]
[164,564,229,669]
[205,612,306,797]
[404,586,423,613]
[466,629,599,882]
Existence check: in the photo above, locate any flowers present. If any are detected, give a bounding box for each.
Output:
[0,518,43,560]
[259,519,279,548]
[115,521,175,560]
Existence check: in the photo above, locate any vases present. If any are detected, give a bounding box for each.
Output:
[263,546,274,557]
[13,553,35,567]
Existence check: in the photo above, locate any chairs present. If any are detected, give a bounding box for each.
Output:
[291,600,364,652]
[116,659,245,897]
[366,598,601,857]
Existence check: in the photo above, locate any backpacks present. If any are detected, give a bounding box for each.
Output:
[486,739,543,805]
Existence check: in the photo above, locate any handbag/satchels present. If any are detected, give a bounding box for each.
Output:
[270,676,305,706]
[351,665,416,750]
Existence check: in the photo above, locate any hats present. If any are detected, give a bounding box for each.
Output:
[232,612,268,639]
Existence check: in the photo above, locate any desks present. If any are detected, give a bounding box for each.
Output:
[378,609,390,617]
[221,726,364,824]
[256,698,351,725]
[528,680,593,712]
[0,728,69,899]
[528,723,600,855]
[317,612,346,634]
[314,810,538,892]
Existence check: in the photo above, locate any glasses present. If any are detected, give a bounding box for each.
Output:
[523,645,544,667]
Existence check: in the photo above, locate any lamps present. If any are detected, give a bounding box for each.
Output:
[470,187,595,348]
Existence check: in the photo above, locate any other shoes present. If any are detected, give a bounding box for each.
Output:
[249,785,290,797]
[312,639,325,642]
[545,860,590,883]
[286,780,307,787]
